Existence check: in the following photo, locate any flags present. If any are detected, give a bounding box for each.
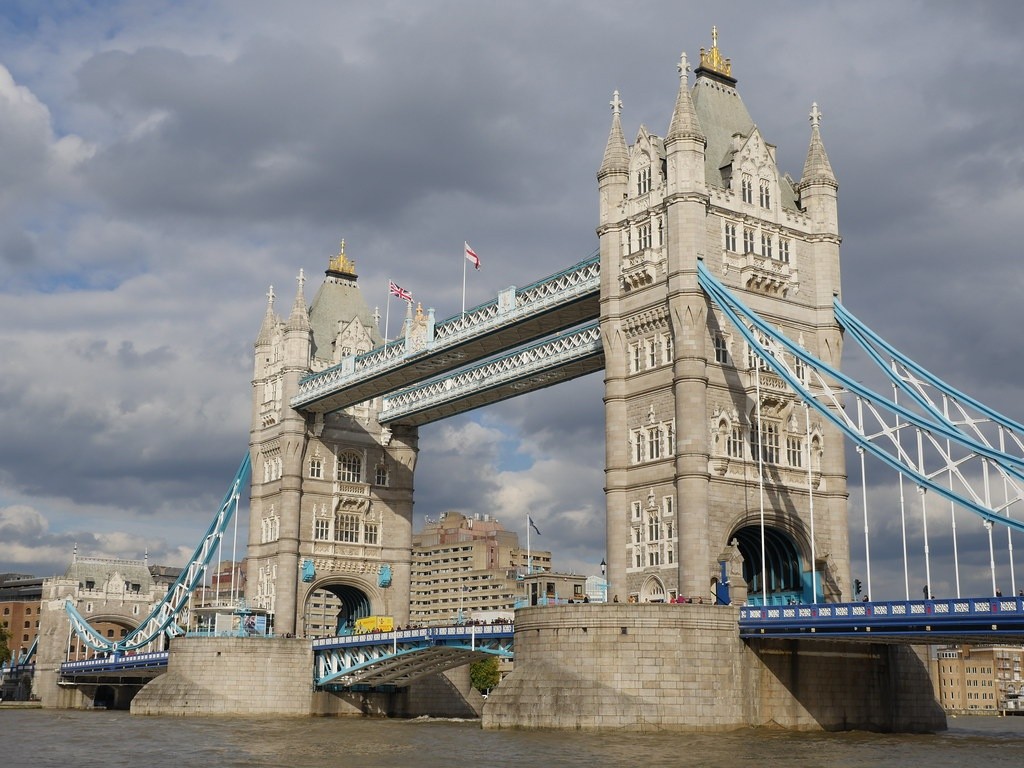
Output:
[465,243,481,271]
[529,516,541,535]
[390,281,414,304]
